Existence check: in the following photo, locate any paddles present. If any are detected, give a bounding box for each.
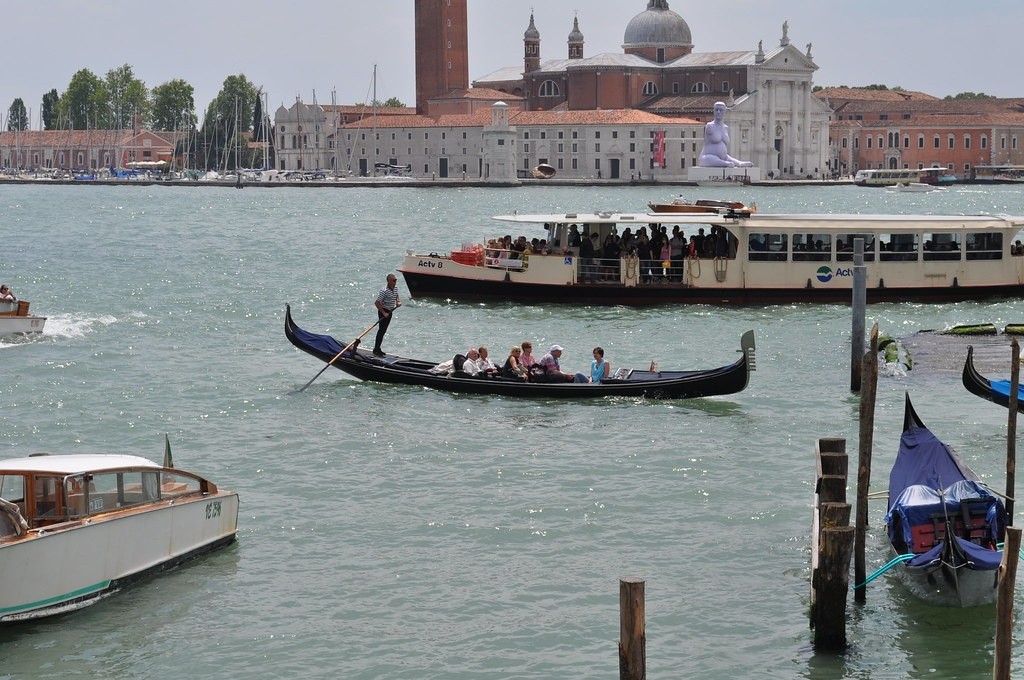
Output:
[296,304,403,393]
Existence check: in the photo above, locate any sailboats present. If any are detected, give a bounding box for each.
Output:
[0,65,416,182]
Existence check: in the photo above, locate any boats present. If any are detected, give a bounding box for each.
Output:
[885,390,1013,608]
[396,197,1024,309]
[969,165,1024,185]
[853,168,956,187]
[0,299,48,336]
[647,194,757,214]
[284,303,757,400]
[961,345,1024,414]
[0,453,240,626]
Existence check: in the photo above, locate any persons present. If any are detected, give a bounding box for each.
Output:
[631,171,641,179]
[373,273,402,357]
[194,172,199,181]
[598,170,601,179]
[699,101,754,168]
[807,174,812,180]
[486,223,727,284]
[822,171,856,181]
[1011,240,1024,256]
[768,171,773,180]
[0,284,18,303]
[463,341,574,383]
[762,235,984,261]
[574,347,610,384]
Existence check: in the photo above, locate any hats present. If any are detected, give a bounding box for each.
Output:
[551,345,564,351]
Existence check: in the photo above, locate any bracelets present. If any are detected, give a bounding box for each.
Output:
[396,300,400,302]
[12,295,14,298]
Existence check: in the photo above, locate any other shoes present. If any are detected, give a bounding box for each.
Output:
[373,351,385,357]
